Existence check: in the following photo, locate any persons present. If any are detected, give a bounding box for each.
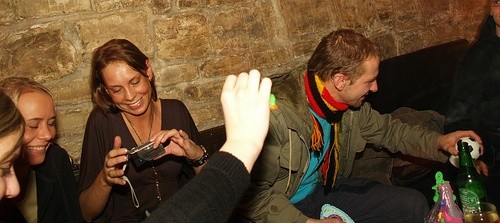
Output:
[0,76,75,223]
[234,29,484,223]
[0,69,271,223]
[444,0,500,207]
[76,39,210,223]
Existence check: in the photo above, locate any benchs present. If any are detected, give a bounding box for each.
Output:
[360,38,471,205]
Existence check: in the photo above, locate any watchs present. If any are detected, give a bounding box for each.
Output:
[185,145,209,167]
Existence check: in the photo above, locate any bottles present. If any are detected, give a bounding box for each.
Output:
[424,183,464,223]
[454,142,489,213]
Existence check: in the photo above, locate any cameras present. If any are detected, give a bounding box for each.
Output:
[129,141,165,168]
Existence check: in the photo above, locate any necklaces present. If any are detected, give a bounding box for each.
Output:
[123,104,154,143]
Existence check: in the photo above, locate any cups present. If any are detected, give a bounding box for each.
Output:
[462,202,500,223]
